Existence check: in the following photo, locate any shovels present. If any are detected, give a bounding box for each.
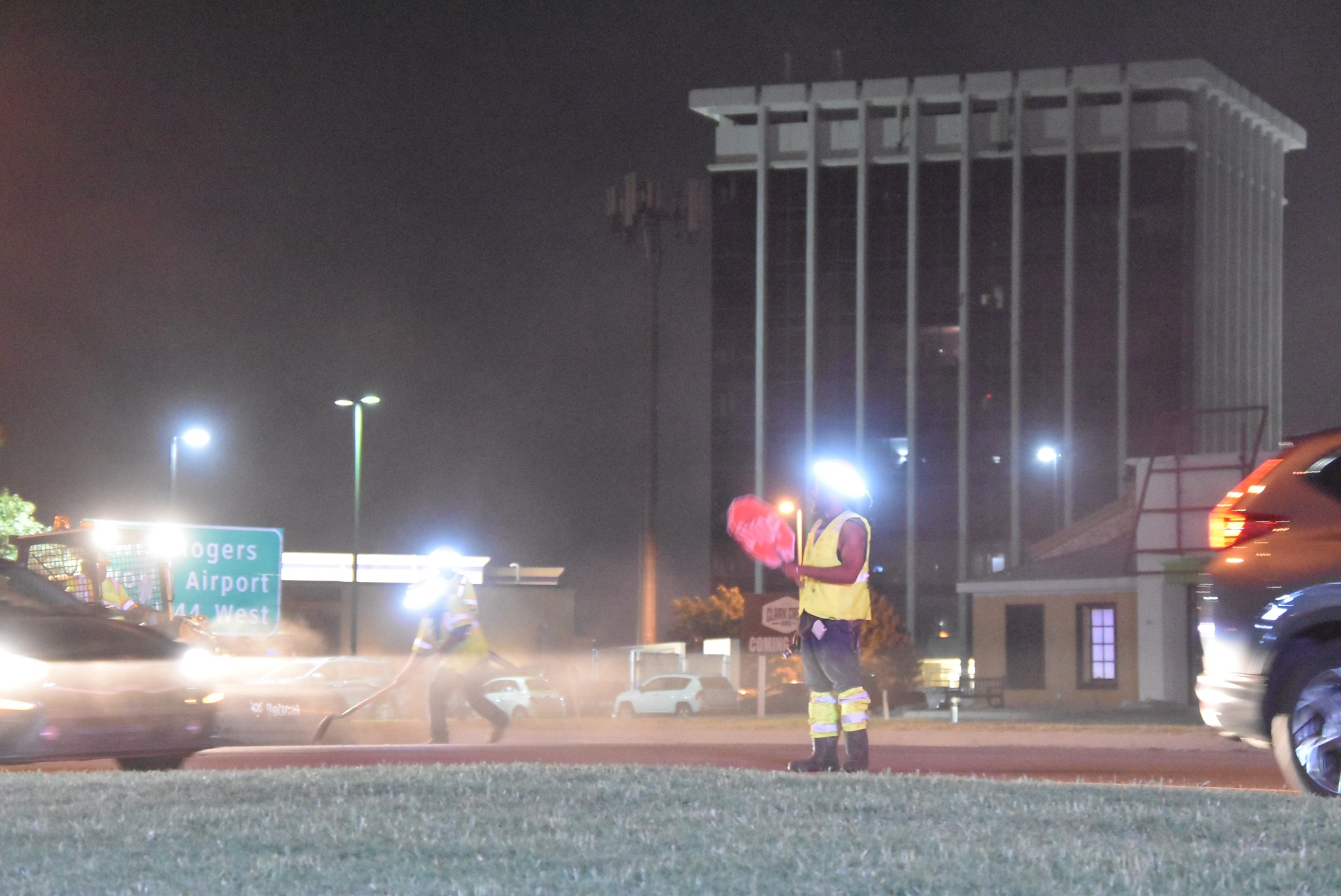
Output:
[307,664,430,745]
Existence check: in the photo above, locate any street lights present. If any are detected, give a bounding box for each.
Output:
[780,501,802,567]
[333,393,380,655]
[509,563,519,582]
[170,426,211,521]
[1038,447,1059,532]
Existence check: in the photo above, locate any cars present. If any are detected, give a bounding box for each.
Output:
[0,558,229,773]
[611,673,738,717]
[457,676,567,719]
[1194,429,1341,798]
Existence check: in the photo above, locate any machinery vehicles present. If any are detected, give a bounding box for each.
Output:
[7,515,229,656]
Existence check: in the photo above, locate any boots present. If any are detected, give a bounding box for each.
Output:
[789,735,841,773]
[843,728,870,773]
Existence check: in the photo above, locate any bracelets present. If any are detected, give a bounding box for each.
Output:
[793,566,801,575]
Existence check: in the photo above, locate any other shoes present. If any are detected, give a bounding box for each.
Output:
[427,733,449,744]
[489,713,508,744]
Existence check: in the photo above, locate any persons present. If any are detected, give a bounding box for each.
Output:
[396,554,512,746]
[782,475,871,774]
[65,554,144,622]
